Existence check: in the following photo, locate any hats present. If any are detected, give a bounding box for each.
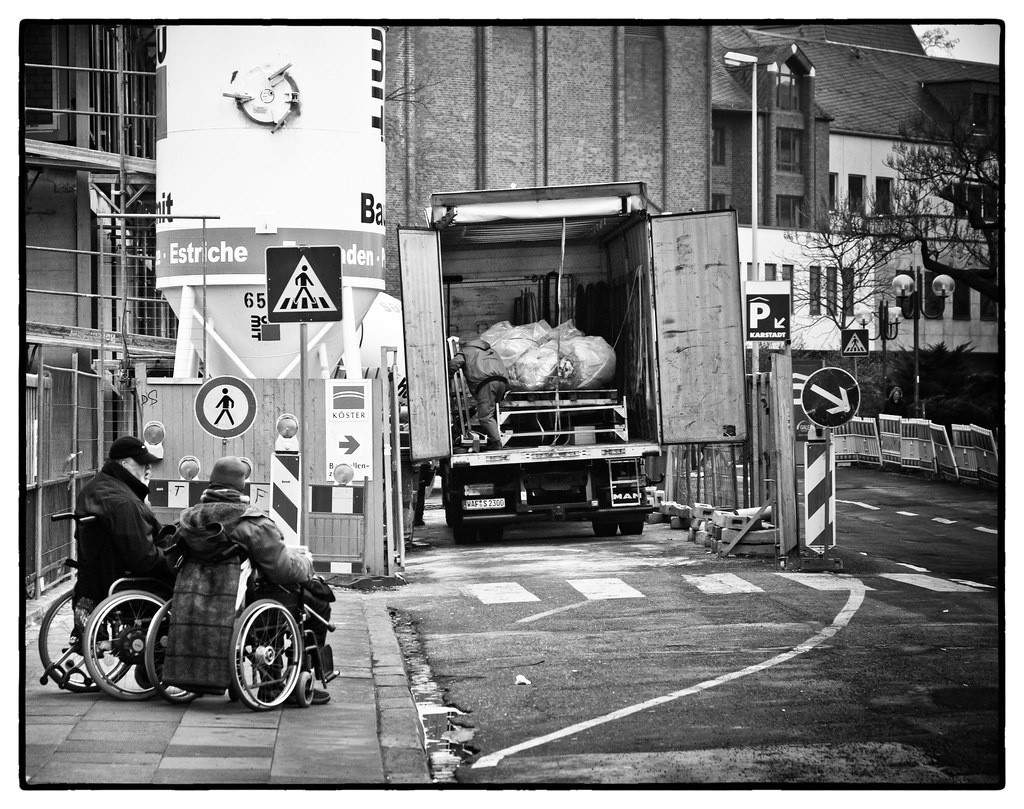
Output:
[208,456,246,491]
[109,435,164,464]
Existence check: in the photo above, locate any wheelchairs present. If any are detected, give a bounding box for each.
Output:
[143,521,341,713]
[34,511,173,711]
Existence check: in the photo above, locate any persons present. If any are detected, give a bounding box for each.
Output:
[71,436,188,689]
[178,455,334,705]
[880,385,910,420]
[447,329,510,452]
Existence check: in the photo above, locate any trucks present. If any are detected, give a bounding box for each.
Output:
[395,178,748,546]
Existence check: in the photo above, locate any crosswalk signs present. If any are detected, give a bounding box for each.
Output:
[264,243,345,323]
[840,328,870,359]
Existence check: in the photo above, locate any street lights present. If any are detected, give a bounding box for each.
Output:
[855,299,904,414]
[721,49,763,373]
[890,264,956,421]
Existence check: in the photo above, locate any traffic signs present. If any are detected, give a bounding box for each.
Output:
[742,277,793,352]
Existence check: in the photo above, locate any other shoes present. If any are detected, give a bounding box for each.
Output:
[481,441,502,450]
[257,682,285,699]
[283,688,330,704]
